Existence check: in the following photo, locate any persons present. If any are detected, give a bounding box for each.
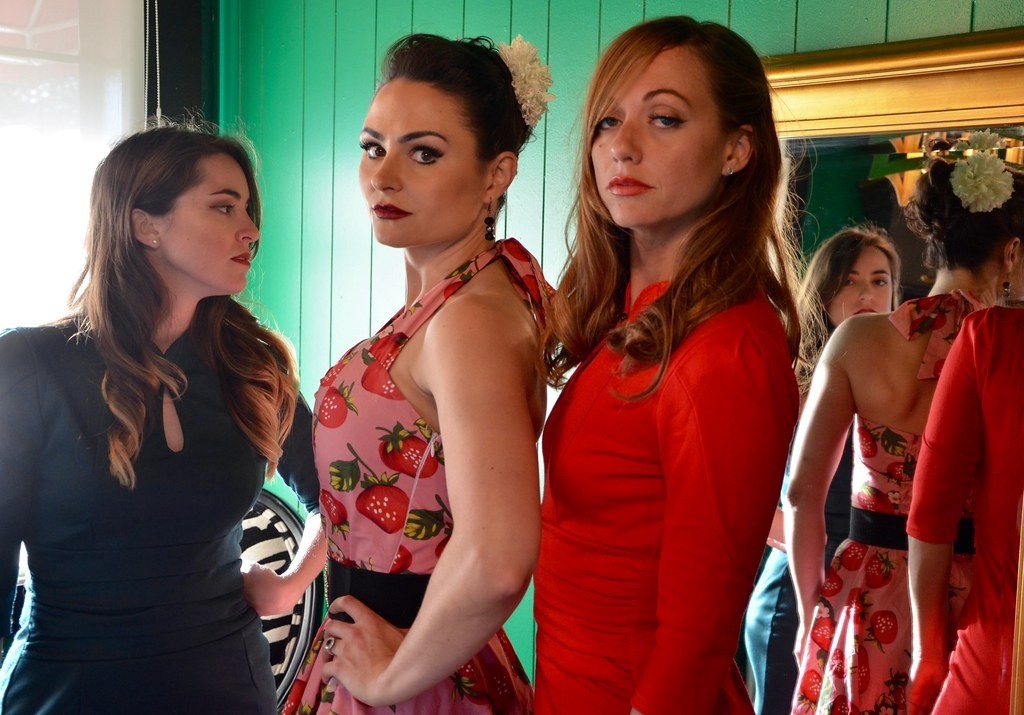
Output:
[528,15,827,715]
[740,150,1024,715]
[275,30,555,714]
[0,120,328,715]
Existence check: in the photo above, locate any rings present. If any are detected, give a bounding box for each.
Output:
[324,637,336,655]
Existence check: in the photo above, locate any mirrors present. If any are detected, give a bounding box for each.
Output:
[736,26,1024,715]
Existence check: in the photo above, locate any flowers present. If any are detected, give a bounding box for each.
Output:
[949,152,1015,213]
[496,33,557,129]
